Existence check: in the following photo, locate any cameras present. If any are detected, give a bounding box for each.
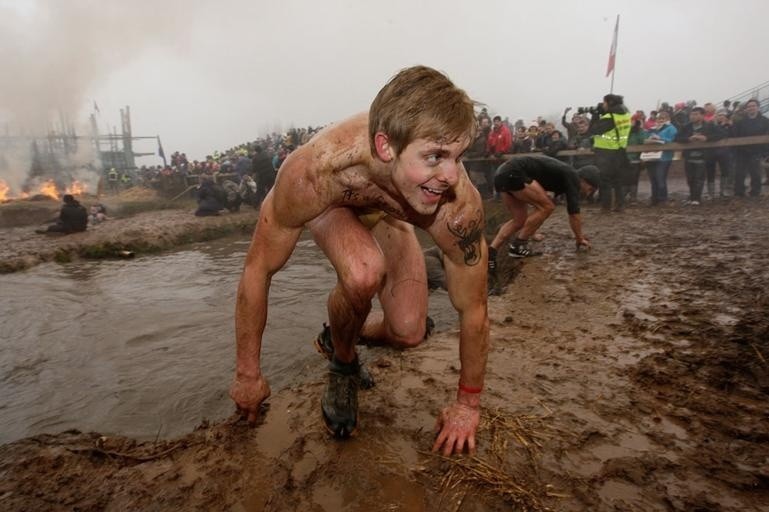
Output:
[578,103,604,114]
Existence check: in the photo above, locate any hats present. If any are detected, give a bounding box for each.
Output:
[571,112,582,119]
[576,164,601,190]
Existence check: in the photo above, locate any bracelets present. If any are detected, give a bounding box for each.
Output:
[459,383,482,393]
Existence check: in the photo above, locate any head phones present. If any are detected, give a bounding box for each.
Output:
[609,100,623,107]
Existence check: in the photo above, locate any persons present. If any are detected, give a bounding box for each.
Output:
[35,195,115,234]
[106,92,768,208]
[425,152,601,292]
[227,66,492,461]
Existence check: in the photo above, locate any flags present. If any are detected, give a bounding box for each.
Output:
[606,16,616,77]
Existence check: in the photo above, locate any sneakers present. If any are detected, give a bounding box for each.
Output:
[508,243,543,258]
[488,254,497,277]
[321,358,361,437]
[314,325,374,390]
[687,200,700,205]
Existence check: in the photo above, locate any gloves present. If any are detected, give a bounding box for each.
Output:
[495,150,502,157]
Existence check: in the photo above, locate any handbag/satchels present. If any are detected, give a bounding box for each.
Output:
[612,147,634,180]
[639,134,666,163]
